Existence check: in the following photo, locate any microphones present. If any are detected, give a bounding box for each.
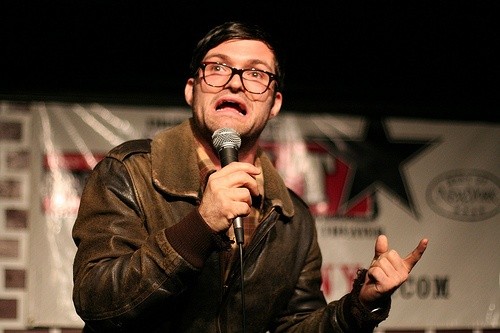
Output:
[212,127,244,245]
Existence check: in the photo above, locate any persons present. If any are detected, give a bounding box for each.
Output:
[68,19,430,332]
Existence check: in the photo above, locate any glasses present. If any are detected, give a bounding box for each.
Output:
[195,60,282,94]
